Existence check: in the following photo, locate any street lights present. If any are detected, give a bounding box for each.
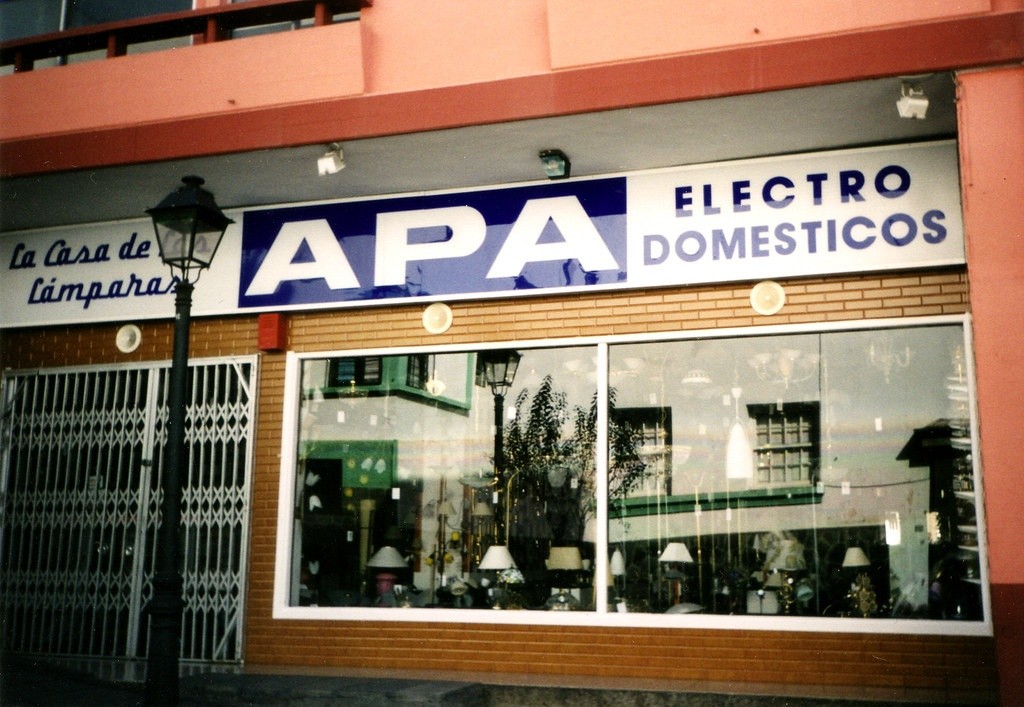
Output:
[480,348,521,547]
[144,173,240,705]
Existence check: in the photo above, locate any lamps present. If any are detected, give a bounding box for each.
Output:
[540,546,585,572]
[436,500,456,515]
[869,340,912,384]
[680,367,713,387]
[427,531,461,586]
[469,502,494,526]
[841,546,871,569]
[564,346,643,389]
[763,573,787,588]
[656,543,694,566]
[477,546,518,572]
[611,547,625,600]
[316,150,347,177]
[766,539,805,571]
[896,84,929,122]
[751,348,821,392]
[362,546,409,572]
[724,384,754,480]
